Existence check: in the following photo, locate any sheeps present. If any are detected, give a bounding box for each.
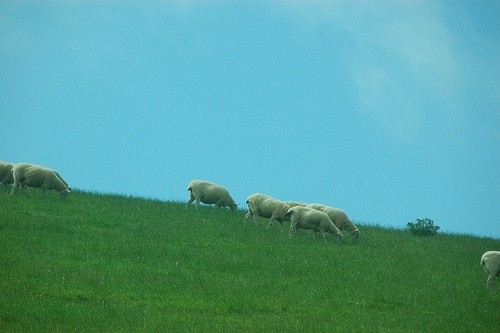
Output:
[244,193,360,246]
[0,160,71,200]
[480,250,500,289]
[185,180,238,214]
